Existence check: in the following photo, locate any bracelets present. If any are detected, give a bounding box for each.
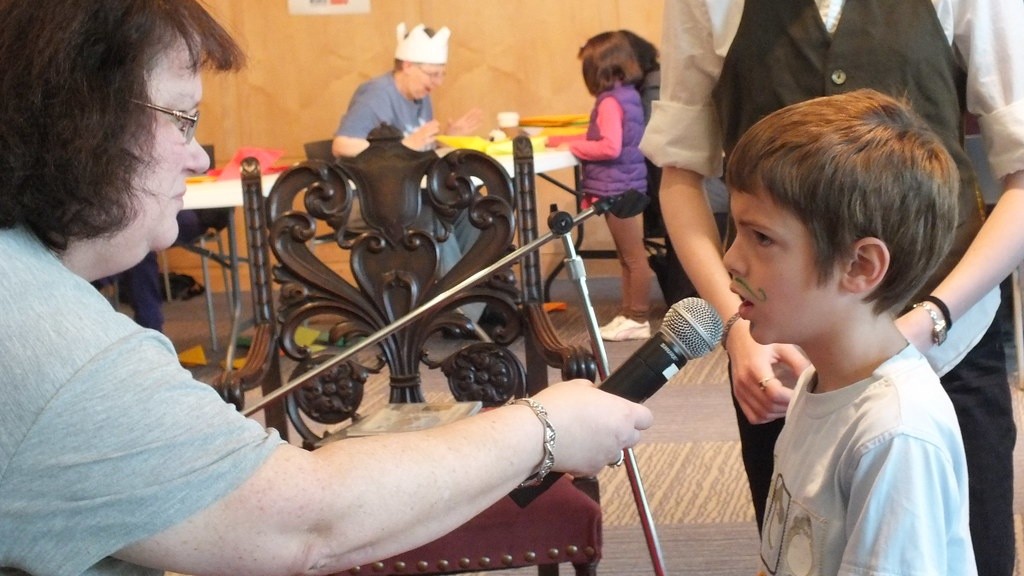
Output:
[719,312,741,350]
[920,296,952,331]
[501,398,556,489]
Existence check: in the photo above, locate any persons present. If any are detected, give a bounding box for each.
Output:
[616,30,729,311]
[635,1,1024,575]
[0,0,653,576]
[331,23,508,341]
[91,208,232,333]
[724,87,979,576]
[545,31,652,341]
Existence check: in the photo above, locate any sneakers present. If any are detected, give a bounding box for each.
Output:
[599,314,652,342]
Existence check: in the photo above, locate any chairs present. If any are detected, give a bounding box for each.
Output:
[238,125,607,576]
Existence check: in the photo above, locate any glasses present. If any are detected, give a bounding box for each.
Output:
[416,63,446,83]
[131,98,201,146]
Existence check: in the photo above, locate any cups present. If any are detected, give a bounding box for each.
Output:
[496,112,519,139]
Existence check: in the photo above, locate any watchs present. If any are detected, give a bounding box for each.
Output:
[911,302,947,347]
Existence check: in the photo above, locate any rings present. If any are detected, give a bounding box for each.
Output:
[608,449,625,469]
[757,377,776,392]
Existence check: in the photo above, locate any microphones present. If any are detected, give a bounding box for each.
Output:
[509,297,724,508]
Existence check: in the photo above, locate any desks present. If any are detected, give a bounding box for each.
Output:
[173,145,664,378]
